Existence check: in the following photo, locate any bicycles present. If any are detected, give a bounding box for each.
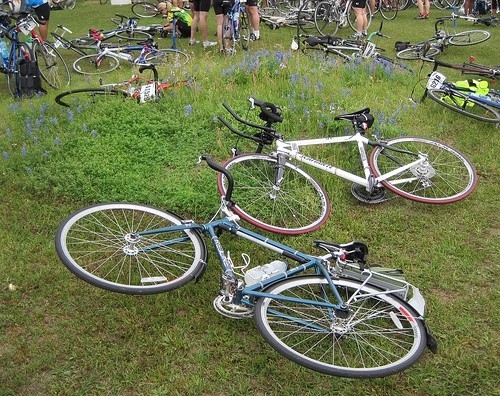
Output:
[220,0,500,123]
[55,153,438,379]
[217,95,479,235]
[0,0,201,108]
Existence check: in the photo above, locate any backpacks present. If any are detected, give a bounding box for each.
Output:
[16,59,41,95]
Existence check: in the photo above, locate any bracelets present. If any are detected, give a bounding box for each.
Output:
[163,28,167,30]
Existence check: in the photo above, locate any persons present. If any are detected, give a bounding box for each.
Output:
[18,0,50,56]
[158,0,268,54]
[414,0,430,20]
[312,0,400,39]
[464,0,500,20]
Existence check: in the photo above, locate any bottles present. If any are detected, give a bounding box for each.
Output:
[119,53,131,59]
[244,260,289,286]
[235,11,239,23]
[233,17,237,27]
[468,92,491,101]
[437,39,442,43]
[0,38,9,59]
[26,38,32,54]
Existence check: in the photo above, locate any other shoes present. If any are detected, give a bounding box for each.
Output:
[414,14,428,20]
[362,30,367,36]
[182,5,192,10]
[243,33,261,41]
[189,39,199,46]
[348,33,362,39]
[203,39,217,48]
[226,48,237,53]
[386,8,389,12]
